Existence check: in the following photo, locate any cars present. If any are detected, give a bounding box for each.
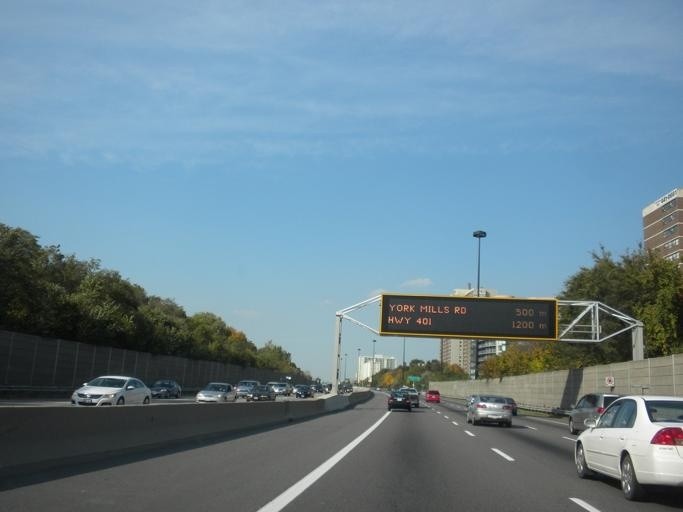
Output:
[312,383,353,395]
[194,381,238,404]
[569,394,625,436]
[425,390,440,402]
[505,397,518,417]
[293,385,301,393]
[466,394,513,427]
[70,375,151,408]
[295,385,315,399]
[247,385,275,402]
[399,388,420,408]
[573,394,682,499]
[388,391,411,412]
[148,379,182,398]
[238,380,261,399]
[264,381,277,392]
[273,383,291,397]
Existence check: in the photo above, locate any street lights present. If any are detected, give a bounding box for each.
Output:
[358,348,361,385]
[344,354,347,381]
[285,376,292,384]
[471,230,487,376]
[372,339,376,386]
